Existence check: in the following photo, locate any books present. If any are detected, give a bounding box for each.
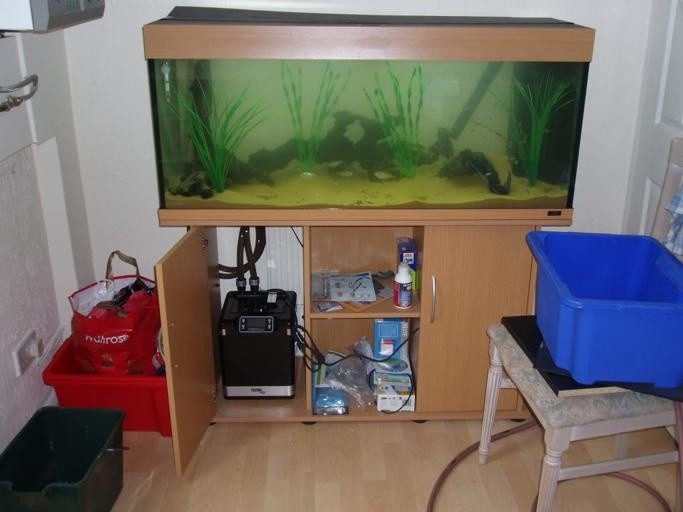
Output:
[338,285,394,312]
[310,271,377,302]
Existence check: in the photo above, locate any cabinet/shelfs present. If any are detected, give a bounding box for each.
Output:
[152,208,574,483]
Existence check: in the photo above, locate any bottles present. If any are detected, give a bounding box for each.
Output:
[392,263,413,310]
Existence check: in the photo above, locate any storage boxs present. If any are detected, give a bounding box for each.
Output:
[2,336,172,511]
[522,226,683,389]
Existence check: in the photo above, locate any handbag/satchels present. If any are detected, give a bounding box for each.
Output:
[67,248,158,377]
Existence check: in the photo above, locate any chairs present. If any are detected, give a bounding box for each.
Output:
[478,137,682,510]
